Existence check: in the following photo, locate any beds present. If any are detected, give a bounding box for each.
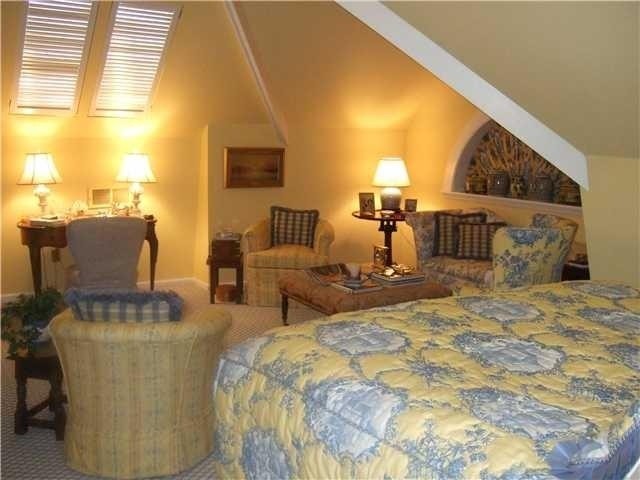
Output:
[214,279,639,479]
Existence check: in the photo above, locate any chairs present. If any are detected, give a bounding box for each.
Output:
[242,218,335,307]
[51,308,231,478]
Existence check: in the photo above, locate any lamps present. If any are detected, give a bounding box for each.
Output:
[114,154,157,211]
[372,158,410,213]
[17,153,63,216]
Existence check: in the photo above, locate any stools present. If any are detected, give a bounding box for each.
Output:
[207,252,242,305]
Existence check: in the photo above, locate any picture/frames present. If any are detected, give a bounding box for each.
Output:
[224,145,285,188]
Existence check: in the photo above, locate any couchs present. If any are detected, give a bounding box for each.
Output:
[406,206,577,288]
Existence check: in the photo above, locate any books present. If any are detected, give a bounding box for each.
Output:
[31,218,65,227]
[370,272,426,287]
[328,278,384,295]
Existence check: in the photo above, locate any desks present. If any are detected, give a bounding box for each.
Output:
[17,215,162,298]
[352,209,407,268]
[6,352,67,442]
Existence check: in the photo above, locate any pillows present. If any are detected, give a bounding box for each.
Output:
[271,206,319,248]
[456,222,506,262]
[433,212,487,256]
[64,286,181,321]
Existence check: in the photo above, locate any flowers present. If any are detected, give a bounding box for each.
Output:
[2,287,61,359]
